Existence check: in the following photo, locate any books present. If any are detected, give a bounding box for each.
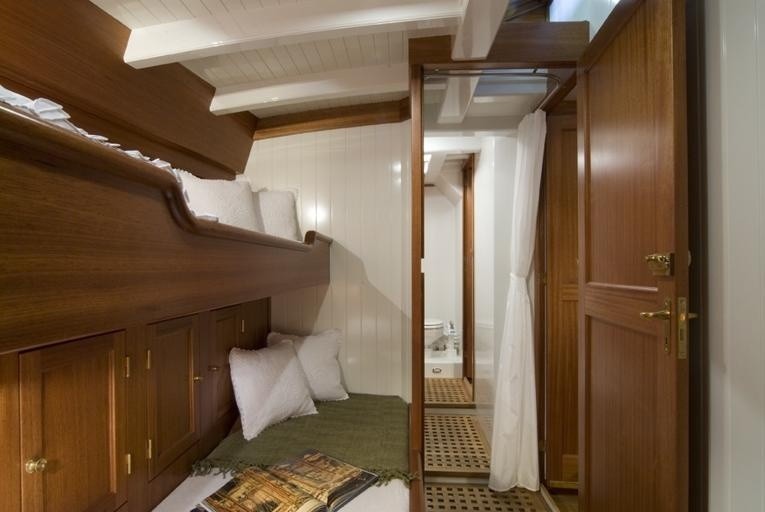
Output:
[190,448,379,512]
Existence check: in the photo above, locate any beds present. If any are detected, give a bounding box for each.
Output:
[144,391,409,512]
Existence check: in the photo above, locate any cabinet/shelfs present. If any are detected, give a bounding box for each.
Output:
[247,298,270,350]
[145,300,246,512]
[18,330,132,512]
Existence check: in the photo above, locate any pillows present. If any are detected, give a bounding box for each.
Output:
[176,167,302,243]
[228,327,350,442]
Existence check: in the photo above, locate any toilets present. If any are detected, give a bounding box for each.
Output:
[425,317,444,348]
